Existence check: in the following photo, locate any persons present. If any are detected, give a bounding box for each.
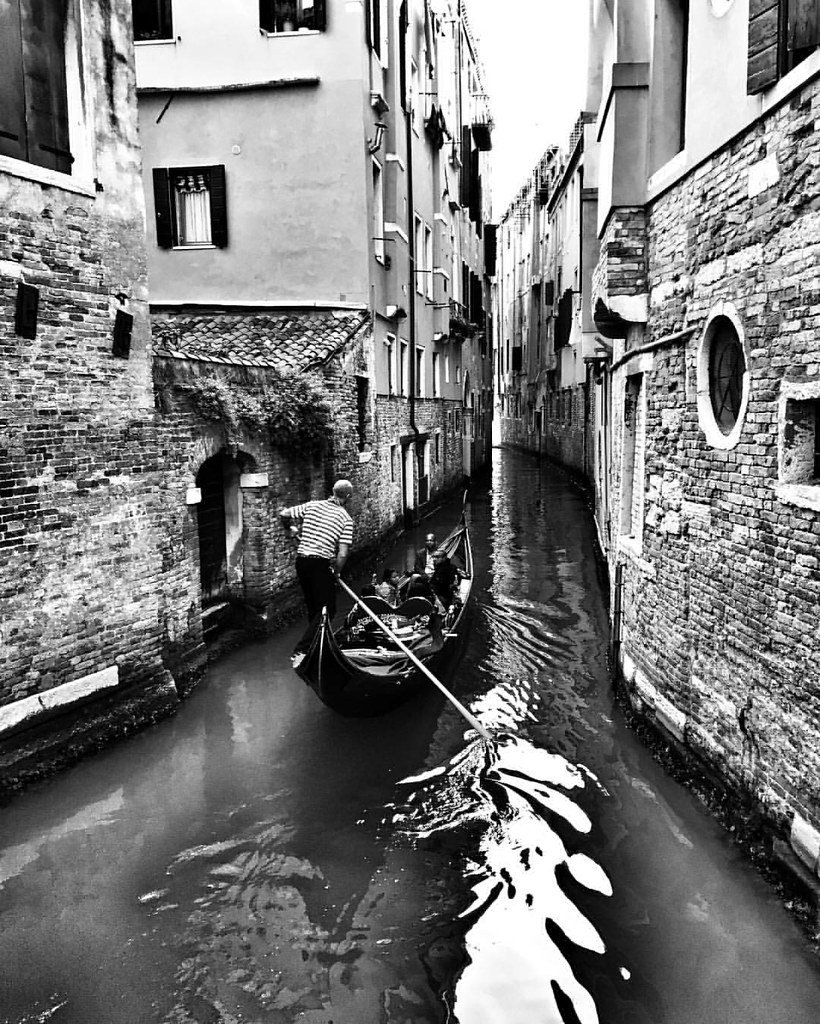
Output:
[360,585,376,598]
[279,480,354,660]
[380,567,402,607]
[429,550,452,595]
[406,574,430,598]
[414,534,447,580]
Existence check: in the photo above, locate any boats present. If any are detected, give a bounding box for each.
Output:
[288,489,477,713]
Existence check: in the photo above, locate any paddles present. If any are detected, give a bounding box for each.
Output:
[291,519,493,741]
